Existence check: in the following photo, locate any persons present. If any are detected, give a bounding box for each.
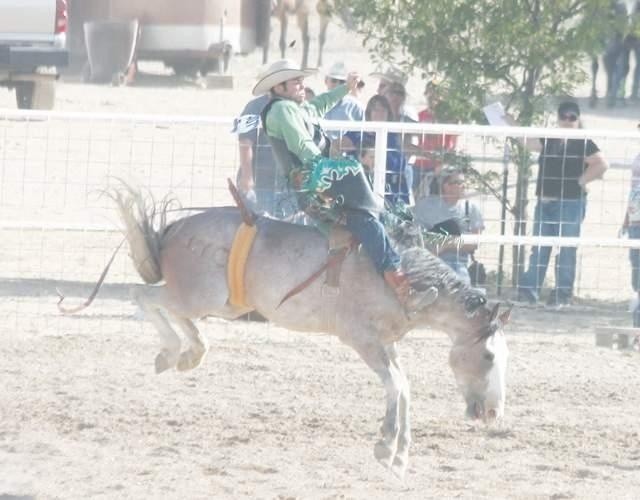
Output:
[236,56,485,314]
[512,99,610,306]
[617,123,640,329]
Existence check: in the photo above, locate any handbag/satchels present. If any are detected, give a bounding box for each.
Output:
[467,261,485,282]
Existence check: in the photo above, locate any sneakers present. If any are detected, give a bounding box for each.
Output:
[545,293,571,310]
[520,272,539,303]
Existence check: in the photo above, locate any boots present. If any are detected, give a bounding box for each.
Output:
[383,266,437,320]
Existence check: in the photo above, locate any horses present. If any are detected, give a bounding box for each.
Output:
[97,175,514,478]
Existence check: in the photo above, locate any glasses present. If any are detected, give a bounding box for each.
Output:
[331,78,345,84]
[559,113,576,121]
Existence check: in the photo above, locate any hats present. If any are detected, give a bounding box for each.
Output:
[251,60,319,96]
[325,63,350,81]
[369,65,407,87]
